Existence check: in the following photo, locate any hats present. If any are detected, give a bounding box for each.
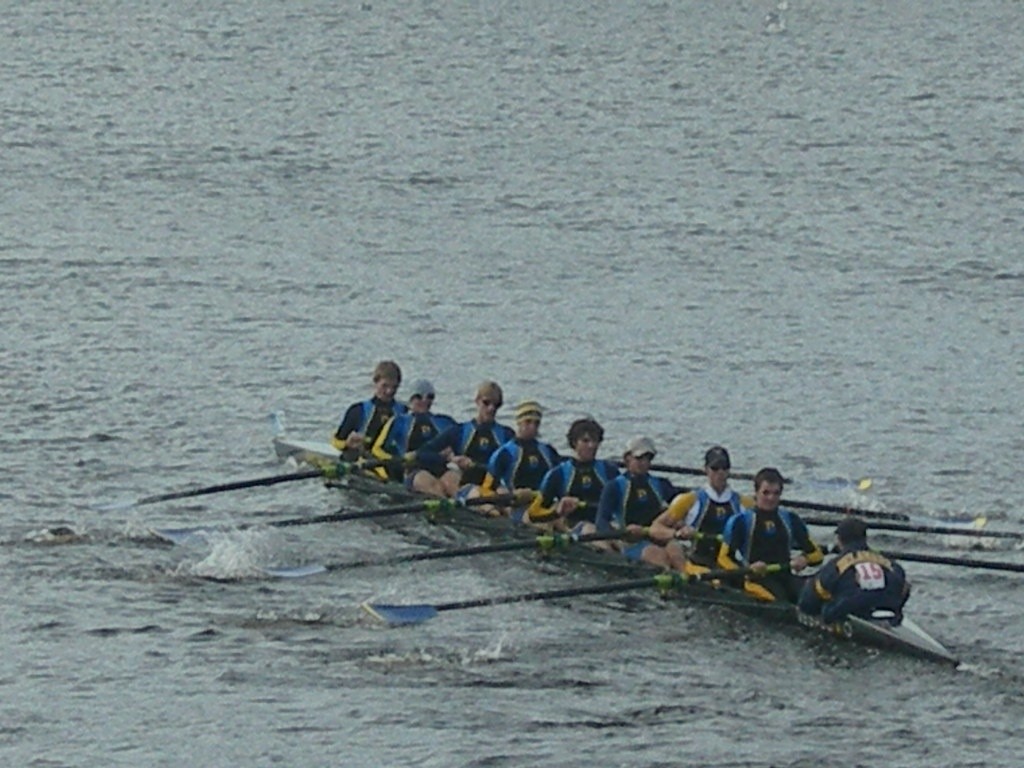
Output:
[834,518,867,541]
[408,378,435,403]
[706,447,729,465]
[516,399,542,424]
[623,436,657,459]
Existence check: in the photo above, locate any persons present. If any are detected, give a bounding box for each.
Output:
[649,446,755,580]
[329,361,515,517]
[530,419,621,549]
[481,401,560,532]
[595,436,678,567]
[719,468,823,601]
[798,518,911,625]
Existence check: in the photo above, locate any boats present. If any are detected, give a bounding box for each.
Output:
[271,434,962,669]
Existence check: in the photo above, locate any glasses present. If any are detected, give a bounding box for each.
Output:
[759,489,780,496]
[482,399,502,409]
[709,465,730,470]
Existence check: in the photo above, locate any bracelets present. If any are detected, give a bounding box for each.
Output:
[674,528,685,539]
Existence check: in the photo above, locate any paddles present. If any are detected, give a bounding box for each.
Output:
[123,459,1023,624]
